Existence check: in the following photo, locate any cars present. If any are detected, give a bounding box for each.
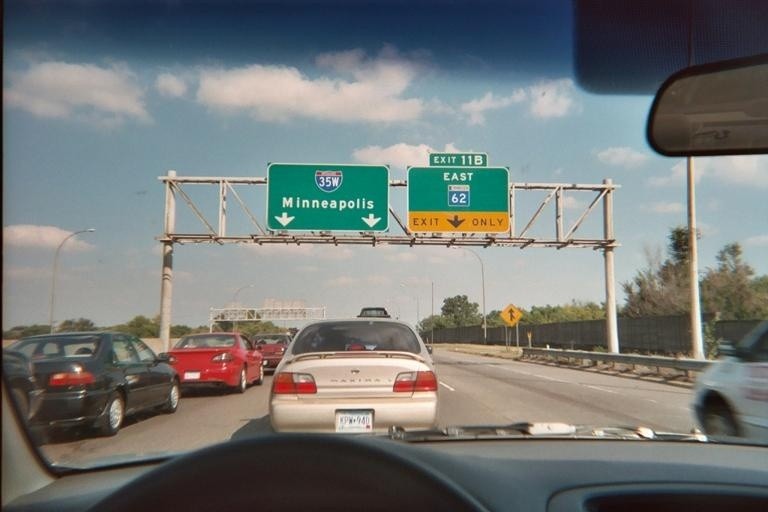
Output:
[267,318,441,437]
[161,332,266,394]
[38,413,766,473]
[10,328,182,440]
[0,348,47,425]
[251,332,293,367]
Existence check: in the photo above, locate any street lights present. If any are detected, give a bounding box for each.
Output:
[399,282,420,335]
[234,284,255,309]
[48,228,97,333]
[452,245,487,346]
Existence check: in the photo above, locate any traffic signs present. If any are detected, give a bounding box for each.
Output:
[405,166,511,235]
[429,152,488,167]
[265,163,390,232]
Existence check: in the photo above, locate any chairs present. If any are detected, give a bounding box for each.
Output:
[318,326,346,352]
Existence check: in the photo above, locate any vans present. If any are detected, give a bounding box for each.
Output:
[692,316,766,442]
